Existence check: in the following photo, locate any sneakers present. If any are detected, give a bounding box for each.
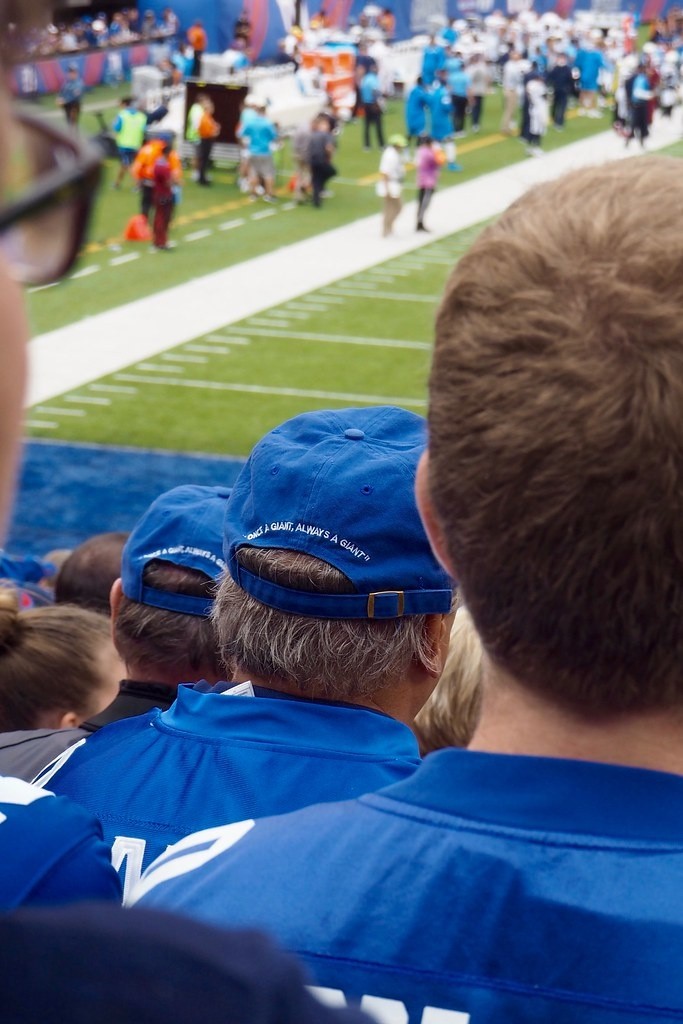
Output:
[447,162,465,173]
[263,194,280,205]
[237,177,245,187]
[249,194,259,202]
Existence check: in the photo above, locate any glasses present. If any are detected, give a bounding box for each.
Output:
[0,112,104,287]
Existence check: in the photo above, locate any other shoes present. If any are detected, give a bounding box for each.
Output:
[383,230,396,243]
[519,135,530,144]
[417,222,430,233]
[364,146,370,153]
[192,169,211,181]
[318,189,334,200]
[500,127,518,138]
[553,123,566,132]
[454,130,468,140]
[109,181,121,191]
[294,199,308,207]
[576,107,604,119]
[133,187,142,196]
[196,181,211,186]
[378,146,387,151]
[472,124,480,133]
[530,147,544,155]
[300,183,309,197]
[152,243,171,253]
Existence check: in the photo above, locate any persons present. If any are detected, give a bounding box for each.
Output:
[120,145,683,1024]
[38,550,72,590]
[0,454,233,786]
[0,0,382,1024]
[413,135,439,233]
[405,0,683,173]
[0,1,406,251]
[1,772,128,909]
[409,606,488,762]
[53,530,130,615]
[29,405,461,909]
[2,588,136,734]
[374,134,408,236]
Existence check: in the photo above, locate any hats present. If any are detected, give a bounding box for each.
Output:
[83,16,92,24]
[17,560,44,584]
[109,21,121,35]
[0,554,26,581]
[257,97,271,108]
[121,483,234,618]
[144,10,154,18]
[245,94,258,107]
[388,133,408,148]
[92,20,106,31]
[222,406,466,618]
[98,12,105,20]
[67,60,79,71]
